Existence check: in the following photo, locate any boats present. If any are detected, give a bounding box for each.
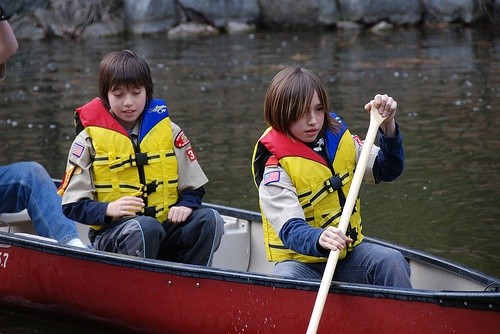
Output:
[0,177,500,334]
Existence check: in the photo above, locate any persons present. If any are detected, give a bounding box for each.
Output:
[56,50,226,267]
[251,64,413,289]
[0,6,86,248]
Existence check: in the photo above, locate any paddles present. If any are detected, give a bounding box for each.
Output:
[304,101,387,333]
